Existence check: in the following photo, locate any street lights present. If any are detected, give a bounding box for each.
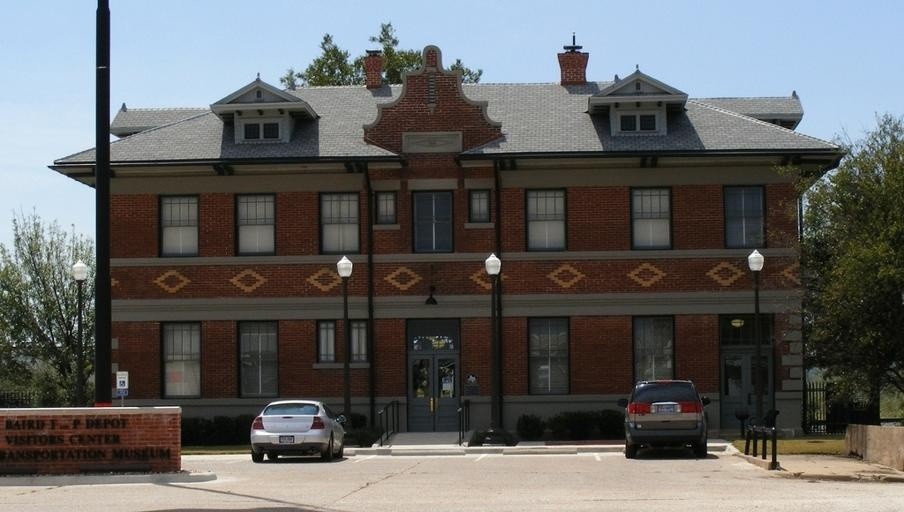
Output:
[332,254,365,441]
[748,247,768,422]
[70,260,92,405]
[483,253,507,445]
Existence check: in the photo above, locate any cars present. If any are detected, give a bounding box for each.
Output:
[250,399,347,462]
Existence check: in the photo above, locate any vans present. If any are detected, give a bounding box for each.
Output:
[615,374,715,463]
